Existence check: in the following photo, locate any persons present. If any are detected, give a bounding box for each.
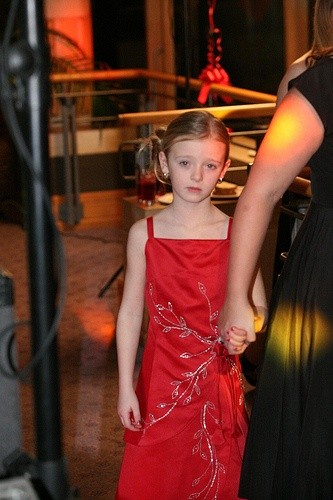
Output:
[115,111,270,500]
[217,1,333,500]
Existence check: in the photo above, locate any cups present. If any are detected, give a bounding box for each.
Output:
[136,164,155,206]
